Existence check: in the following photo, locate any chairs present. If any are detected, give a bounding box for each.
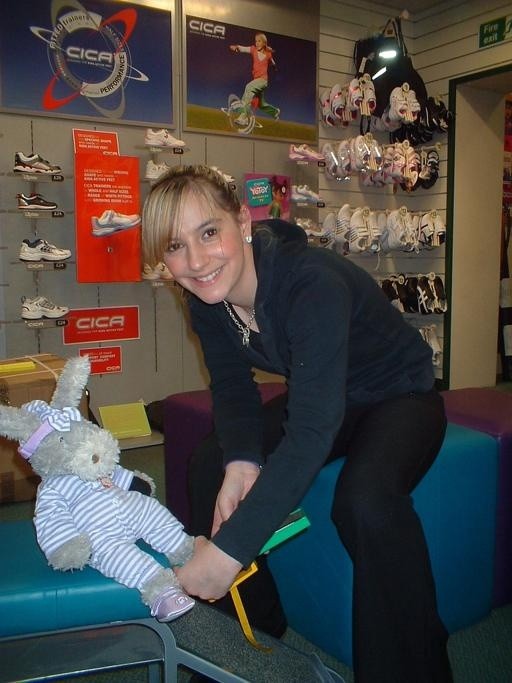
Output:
[0,511,243,683]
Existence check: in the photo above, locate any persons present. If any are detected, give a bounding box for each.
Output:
[225,34,281,127]
[138,165,449,680]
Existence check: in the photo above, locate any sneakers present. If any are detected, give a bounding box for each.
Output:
[293,218,324,236]
[273,108,281,123]
[389,96,450,146]
[381,86,421,132]
[92,210,141,236]
[147,588,196,624]
[366,208,446,255]
[322,142,338,180]
[142,261,174,281]
[19,294,69,320]
[320,87,334,127]
[13,150,63,175]
[234,115,249,125]
[18,239,72,262]
[145,160,169,179]
[379,272,449,316]
[144,128,186,149]
[349,208,369,255]
[331,73,380,130]
[291,185,320,204]
[287,143,324,162]
[428,324,443,367]
[321,211,335,250]
[335,203,350,241]
[336,140,442,193]
[16,191,57,210]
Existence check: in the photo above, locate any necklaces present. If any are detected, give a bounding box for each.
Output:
[222,296,264,348]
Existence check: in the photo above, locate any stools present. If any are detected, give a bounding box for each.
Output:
[438,382,511,608]
[265,418,499,654]
[163,381,289,544]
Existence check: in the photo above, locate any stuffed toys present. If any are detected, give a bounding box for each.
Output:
[3,354,199,623]
